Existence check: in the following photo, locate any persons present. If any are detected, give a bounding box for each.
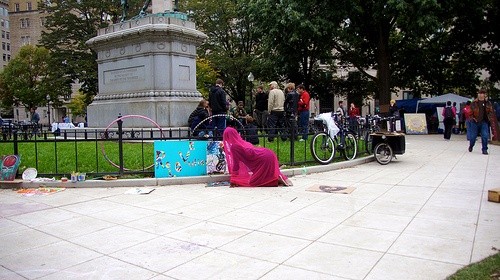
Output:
[388,99,399,131]
[223,127,294,187]
[254,82,311,142]
[349,103,360,132]
[187,96,260,145]
[209,78,229,140]
[442,100,478,141]
[30,107,41,138]
[337,100,347,118]
[64,116,69,123]
[467,88,495,156]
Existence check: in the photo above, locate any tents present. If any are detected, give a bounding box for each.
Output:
[394,93,472,129]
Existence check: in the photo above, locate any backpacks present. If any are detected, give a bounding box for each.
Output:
[444,106,453,119]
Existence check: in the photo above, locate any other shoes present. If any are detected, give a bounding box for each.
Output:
[482,150,488,154]
[469,146,472,152]
[299,139,305,142]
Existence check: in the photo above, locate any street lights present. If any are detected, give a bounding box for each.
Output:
[248,72,254,118]
[46,94,52,131]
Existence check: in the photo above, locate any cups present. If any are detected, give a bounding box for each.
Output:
[71,173,85,183]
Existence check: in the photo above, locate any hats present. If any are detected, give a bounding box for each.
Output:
[477,89,487,93]
[268,81,277,85]
[390,99,395,103]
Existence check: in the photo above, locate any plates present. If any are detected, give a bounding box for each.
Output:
[22,168,37,181]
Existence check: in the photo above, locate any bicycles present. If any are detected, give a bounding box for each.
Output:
[310,116,358,165]
[360,115,403,165]
